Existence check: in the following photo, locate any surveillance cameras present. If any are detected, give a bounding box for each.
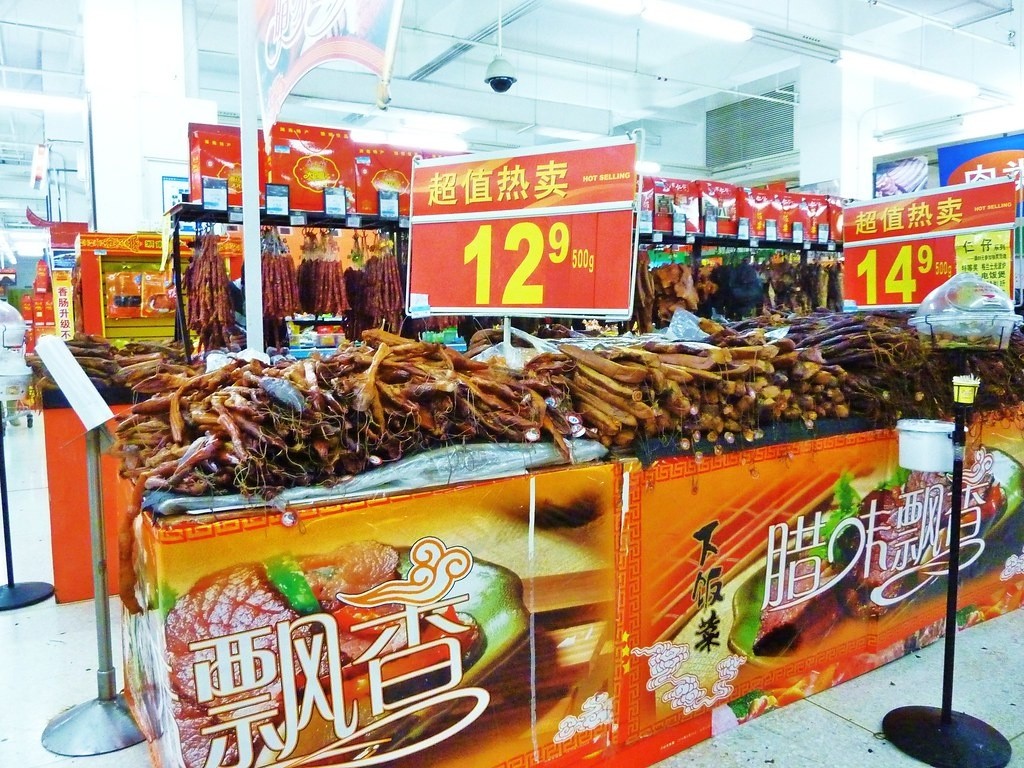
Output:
[484,55,518,92]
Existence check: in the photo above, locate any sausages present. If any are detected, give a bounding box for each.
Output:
[185,234,463,359]
